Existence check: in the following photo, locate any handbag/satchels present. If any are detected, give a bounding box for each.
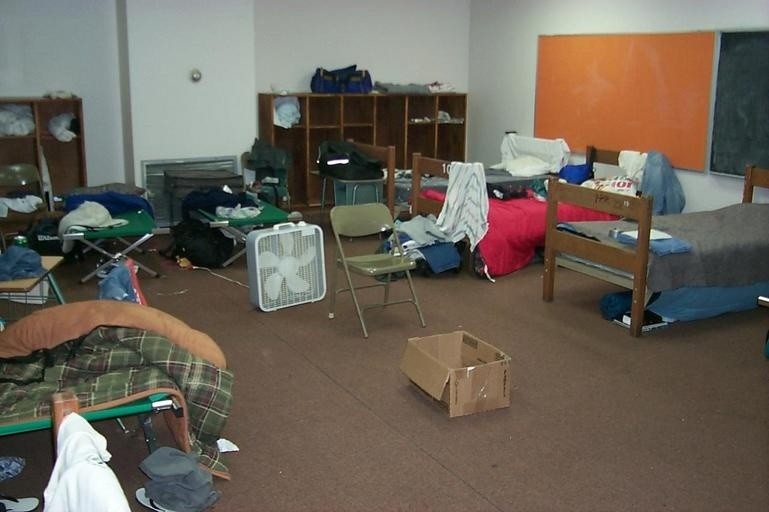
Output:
[29,218,84,265]
[317,140,385,180]
[311,65,372,93]
[173,220,233,268]
[559,164,594,185]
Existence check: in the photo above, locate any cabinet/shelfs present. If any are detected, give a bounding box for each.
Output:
[0,96,86,244]
[258,91,469,211]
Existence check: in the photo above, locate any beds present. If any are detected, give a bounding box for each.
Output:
[542,161,769,337]
[411,145,686,276]
[345,130,571,218]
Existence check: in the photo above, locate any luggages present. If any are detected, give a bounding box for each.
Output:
[247,183,290,212]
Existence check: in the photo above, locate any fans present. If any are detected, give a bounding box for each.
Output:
[245,221,327,314]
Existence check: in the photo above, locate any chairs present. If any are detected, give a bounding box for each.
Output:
[0,163,65,251]
[328,203,426,338]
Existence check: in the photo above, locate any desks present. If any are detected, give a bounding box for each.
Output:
[61,209,160,285]
[310,170,386,216]
[0,386,185,457]
[197,192,302,267]
[162,168,245,229]
[0,255,68,321]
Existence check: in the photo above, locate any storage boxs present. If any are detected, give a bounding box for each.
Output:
[399,330,513,419]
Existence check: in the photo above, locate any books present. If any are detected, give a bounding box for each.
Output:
[613,309,680,332]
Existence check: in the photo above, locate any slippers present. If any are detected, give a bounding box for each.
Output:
[136,488,173,512]
[0,496,39,512]
